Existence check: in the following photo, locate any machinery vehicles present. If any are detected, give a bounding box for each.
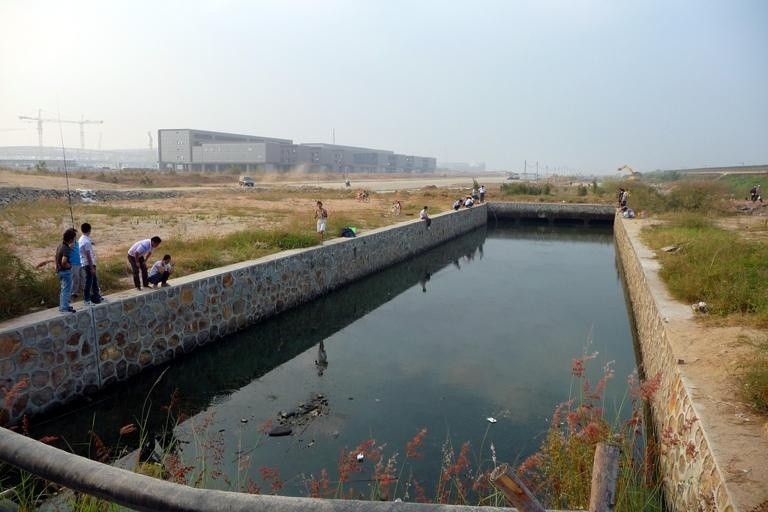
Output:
[618,164,643,182]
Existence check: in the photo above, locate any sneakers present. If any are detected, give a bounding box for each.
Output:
[84,297,104,307]
[59,306,76,313]
[138,283,170,291]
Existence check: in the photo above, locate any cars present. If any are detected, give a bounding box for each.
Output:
[239,176,255,187]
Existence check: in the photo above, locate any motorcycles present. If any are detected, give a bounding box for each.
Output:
[357,190,370,203]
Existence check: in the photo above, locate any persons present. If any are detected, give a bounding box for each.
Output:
[128,237,161,291]
[420,206,431,230]
[420,276,430,293]
[750,185,763,203]
[314,340,328,377]
[78,223,104,305]
[346,177,350,188]
[55,230,76,312]
[618,188,635,218]
[148,254,174,290]
[452,186,486,210]
[313,202,327,244]
[68,229,83,298]
